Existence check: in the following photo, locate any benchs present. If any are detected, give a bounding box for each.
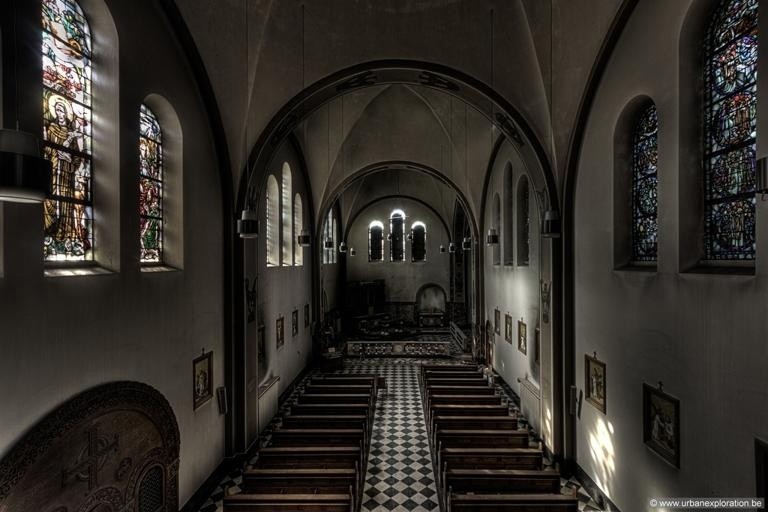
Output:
[222,372,388,512]
[419,361,580,511]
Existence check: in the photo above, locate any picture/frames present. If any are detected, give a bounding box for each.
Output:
[583,350,607,416]
[641,381,682,473]
[192,301,315,412]
[494,308,540,362]
[753,434,768,511]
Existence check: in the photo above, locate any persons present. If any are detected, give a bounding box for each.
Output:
[43,102,90,258]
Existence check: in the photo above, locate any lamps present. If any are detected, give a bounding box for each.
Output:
[448,243,457,253]
[487,229,499,247]
[325,237,333,249]
[298,230,311,247]
[462,238,472,250]
[541,210,559,239]
[440,243,447,253]
[350,248,356,256]
[339,242,346,252]
[240,211,260,238]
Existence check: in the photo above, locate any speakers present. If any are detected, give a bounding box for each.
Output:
[488,328,493,344]
[310,322,316,335]
[569,386,577,416]
[217,386,228,414]
